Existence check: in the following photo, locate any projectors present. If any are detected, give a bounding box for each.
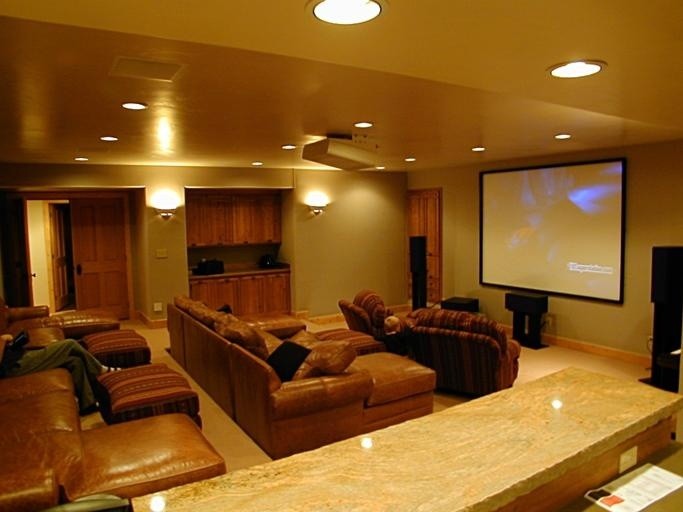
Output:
[302,135,379,170]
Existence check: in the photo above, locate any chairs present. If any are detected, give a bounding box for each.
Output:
[337,288,411,340]
[402,308,522,399]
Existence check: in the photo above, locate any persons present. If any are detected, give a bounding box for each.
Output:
[0,332,125,417]
[381,315,409,359]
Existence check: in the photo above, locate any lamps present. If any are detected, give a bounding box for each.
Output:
[301,187,331,214]
[144,184,181,217]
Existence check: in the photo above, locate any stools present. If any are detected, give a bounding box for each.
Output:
[314,327,386,358]
[94,362,202,430]
[83,329,150,368]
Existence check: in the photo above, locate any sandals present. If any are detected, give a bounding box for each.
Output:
[107,367,121,373]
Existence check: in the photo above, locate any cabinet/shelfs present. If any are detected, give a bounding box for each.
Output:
[208,196,232,246]
[239,274,262,316]
[191,278,213,307]
[232,196,255,245]
[213,275,238,318]
[262,270,289,313]
[255,196,281,244]
[405,190,440,237]
[405,237,440,307]
[186,196,208,246]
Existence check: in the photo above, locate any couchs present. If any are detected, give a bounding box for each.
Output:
[163,295,438,460]
[0,304,226,511]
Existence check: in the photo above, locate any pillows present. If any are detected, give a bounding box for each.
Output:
[172,295,354,383]
